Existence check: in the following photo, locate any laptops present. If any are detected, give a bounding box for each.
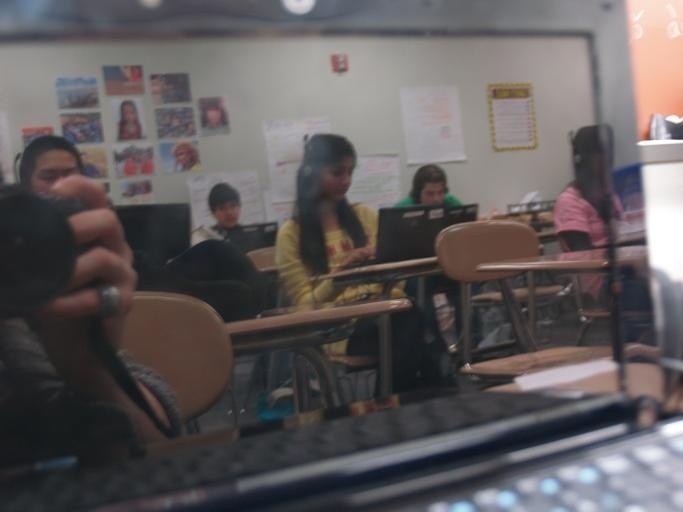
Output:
[446,203,478,224]
[338,204,447,270]
[231,222,277,253]
[1,0,683,512]
[114,203,190,289]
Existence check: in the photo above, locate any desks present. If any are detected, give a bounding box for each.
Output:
[222,257,455,432]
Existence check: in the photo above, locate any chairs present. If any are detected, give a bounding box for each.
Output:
[246,248,282,280]
[95,289,235,440]
[436,201,651,379]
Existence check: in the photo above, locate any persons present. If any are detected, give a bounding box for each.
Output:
[200,101,227,129]
[117,100,145,140]
[0,172,181,461]
[171,144,203,169]
[272,132,468,391]
[555,122,650,311]
[18,138,82,202]
[120,64,142,81]
[387,163,483,352]
[197,181,246,235]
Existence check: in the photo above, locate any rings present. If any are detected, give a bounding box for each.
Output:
[97,287,122,310]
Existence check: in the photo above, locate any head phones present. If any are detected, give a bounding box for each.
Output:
[301,164,314,179]
[572,153,584,165]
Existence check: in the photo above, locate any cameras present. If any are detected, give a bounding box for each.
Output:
[1,185,91,319]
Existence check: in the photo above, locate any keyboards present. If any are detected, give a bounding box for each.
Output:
[303,134,309,164]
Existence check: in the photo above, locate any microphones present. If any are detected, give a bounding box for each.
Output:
[13,152,21,183]
[569,129,576,152]
